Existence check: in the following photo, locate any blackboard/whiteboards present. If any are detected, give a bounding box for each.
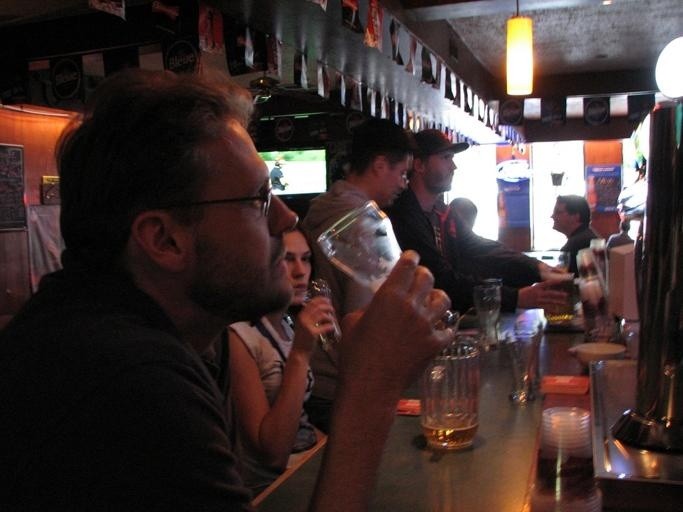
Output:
[0,143,28,232]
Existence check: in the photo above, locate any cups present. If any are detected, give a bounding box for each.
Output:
[493,161,530,229]
[527,407,605,511]
[418,344,481,450]
[301,280,340,348]
[472,276,542,403]
[316,200,453,332]
[543,275,571,315]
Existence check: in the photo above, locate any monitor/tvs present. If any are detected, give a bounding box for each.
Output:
[254,145,331,202]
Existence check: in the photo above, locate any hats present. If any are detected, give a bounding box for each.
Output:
[412,128,470,162]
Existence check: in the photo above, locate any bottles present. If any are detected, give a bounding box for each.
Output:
[574,238,611,342]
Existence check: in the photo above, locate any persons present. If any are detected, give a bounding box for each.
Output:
[605,220,633,247]
[447,197,478,230]
[548,196,603,274]
[299,119,416,317]
[388,127,572,313]
[270,158,288,193]
[0,67,456,511]
[213,225,341,482]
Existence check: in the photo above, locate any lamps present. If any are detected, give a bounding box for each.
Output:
[504,0,536,98]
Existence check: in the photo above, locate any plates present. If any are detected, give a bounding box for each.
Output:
[548,319,569,328]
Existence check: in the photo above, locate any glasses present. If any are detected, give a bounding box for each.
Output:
[149,176,273,217]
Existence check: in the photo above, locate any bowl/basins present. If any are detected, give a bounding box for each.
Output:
[568,343,626,369]
